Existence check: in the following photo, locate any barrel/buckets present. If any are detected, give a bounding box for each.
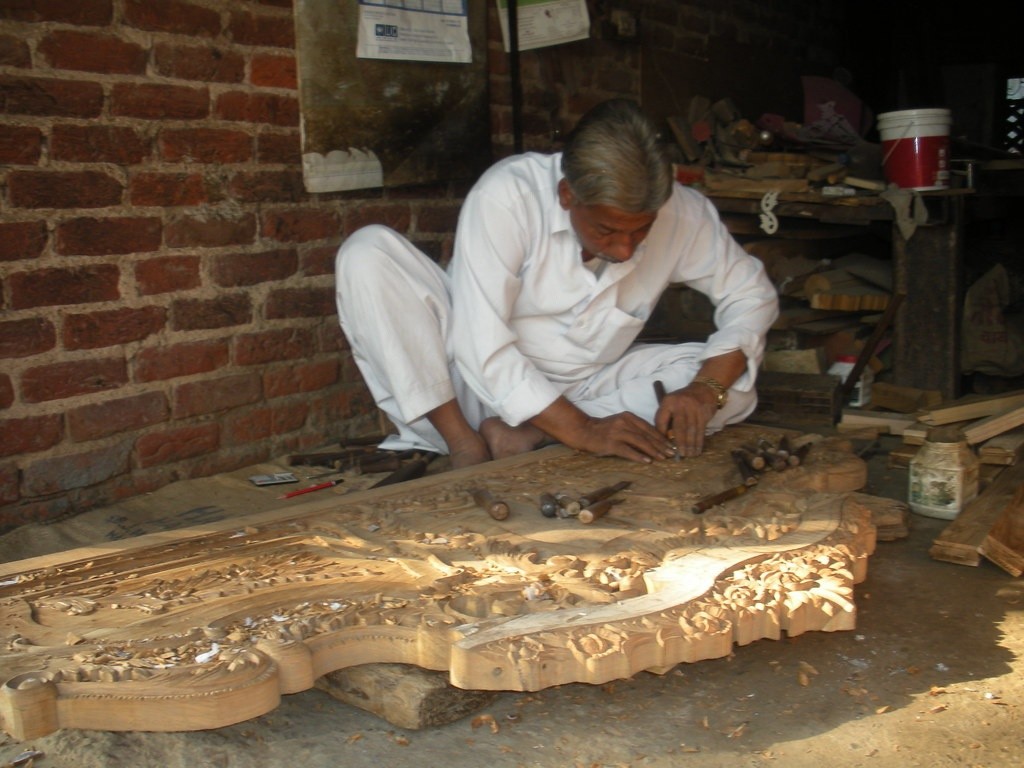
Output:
[877,108,952,191]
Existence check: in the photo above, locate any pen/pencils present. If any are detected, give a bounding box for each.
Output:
[275,478,346,500]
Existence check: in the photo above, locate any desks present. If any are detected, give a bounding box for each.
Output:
[709,185,990,400]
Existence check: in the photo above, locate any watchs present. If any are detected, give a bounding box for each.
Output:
[692,375,728,409]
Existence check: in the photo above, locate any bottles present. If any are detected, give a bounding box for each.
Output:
[907,428,980,520]
[827,354,874,407]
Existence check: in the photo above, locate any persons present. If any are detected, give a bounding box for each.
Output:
[334,99,780,474]
